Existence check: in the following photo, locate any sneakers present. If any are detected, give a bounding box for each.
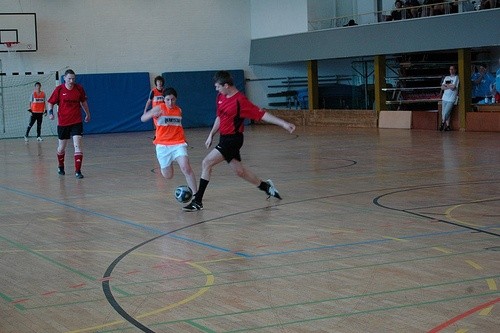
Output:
[266,179,283,202]
[182,198,204,212]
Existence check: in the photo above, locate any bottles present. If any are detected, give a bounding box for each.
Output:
[492,96,496,104]
[485,96,488,103]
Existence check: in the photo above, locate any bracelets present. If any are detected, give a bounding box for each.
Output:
[45,110,47,113]
[47,109,53,115]
[29,109,31,112]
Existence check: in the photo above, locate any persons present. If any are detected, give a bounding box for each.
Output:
[144,76,167,142]
[180,70,296,212]
[472,63,500,103]
[47,68,90,179]
[383,0,500,24]
[141,87,197,197]
[343,19,358,26]
[439,66,459,131]
[23,82,47,142]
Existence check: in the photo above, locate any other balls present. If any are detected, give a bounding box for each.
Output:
[174,186,194,203]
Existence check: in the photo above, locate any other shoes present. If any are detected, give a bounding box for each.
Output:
[58,165,65,175]
[439,122,446,130]
[37,136,43,141]
[74,170,84,179]
[24,134,29,142]
[444,125,451,132]
